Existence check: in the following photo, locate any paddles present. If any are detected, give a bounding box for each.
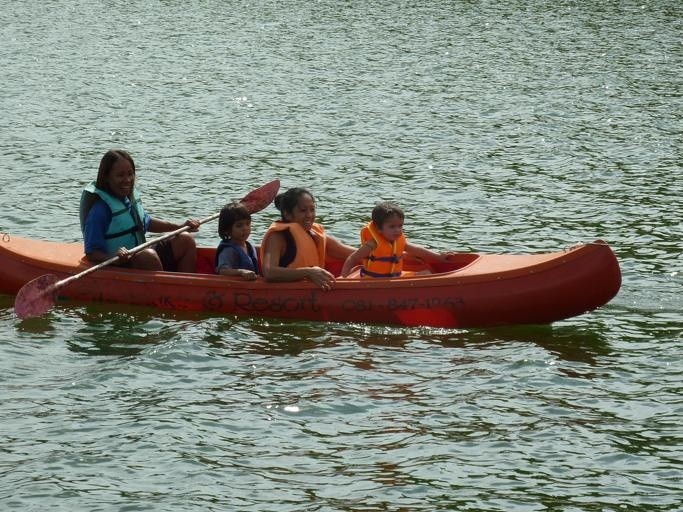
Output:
[14,178,280,320]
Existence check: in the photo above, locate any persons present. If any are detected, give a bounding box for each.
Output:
[79,150,201,271]
[214,202,262,280]
[258,187,360,292]
[340,202,456,279]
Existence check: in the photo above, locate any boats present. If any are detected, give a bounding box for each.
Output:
[0,232,621,327]
